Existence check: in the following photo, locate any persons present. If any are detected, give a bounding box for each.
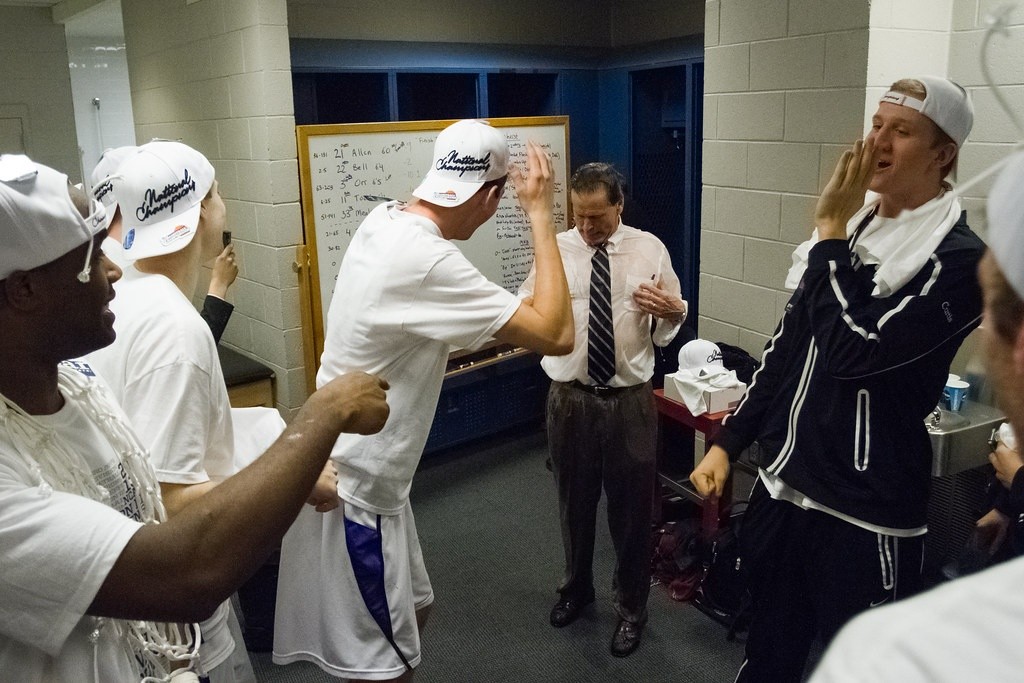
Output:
[809,153,1024,683]
[81,139,339,683]
[691,76,987,683]
[518,163,688,657]
[0,155,389,683]
[975,440,1024,564]
[273,120,577,683]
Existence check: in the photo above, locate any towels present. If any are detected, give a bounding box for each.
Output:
[785,180,961,299]
[673,370,740,417]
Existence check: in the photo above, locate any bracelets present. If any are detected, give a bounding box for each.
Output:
[669,306,686,323]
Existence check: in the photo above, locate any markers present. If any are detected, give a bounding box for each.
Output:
[497,347,521,358]
[451,362,474,371]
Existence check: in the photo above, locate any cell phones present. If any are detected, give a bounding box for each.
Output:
[222,231,231,249]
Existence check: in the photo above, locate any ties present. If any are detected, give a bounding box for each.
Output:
[587,244,616,386]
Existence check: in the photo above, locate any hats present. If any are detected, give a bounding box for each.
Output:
[89,145,140,231]
[880,76,973,183]
[117,141,215,260]
[986,152,1024,296]
[0,154,110,283]
[412,119,511,207]
[678,339,731,380]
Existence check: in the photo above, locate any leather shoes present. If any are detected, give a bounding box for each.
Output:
[550,586,595,627]
[610,619,643,657]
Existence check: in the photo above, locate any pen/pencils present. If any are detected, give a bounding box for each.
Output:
[650,273,655,280]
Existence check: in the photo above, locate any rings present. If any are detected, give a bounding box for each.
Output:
[652,303,656,309]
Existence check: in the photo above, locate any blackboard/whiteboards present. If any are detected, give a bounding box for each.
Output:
[295,114,571,397]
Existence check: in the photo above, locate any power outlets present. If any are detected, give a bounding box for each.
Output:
[749,440,758,466]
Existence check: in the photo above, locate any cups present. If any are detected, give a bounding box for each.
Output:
[945,381,970,412]
[939,374,960,407]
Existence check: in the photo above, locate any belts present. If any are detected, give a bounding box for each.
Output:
[569,379,645,398]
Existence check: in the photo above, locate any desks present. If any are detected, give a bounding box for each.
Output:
[922,401,1009,561]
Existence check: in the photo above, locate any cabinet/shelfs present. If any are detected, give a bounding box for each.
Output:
[654,389,757,575]
